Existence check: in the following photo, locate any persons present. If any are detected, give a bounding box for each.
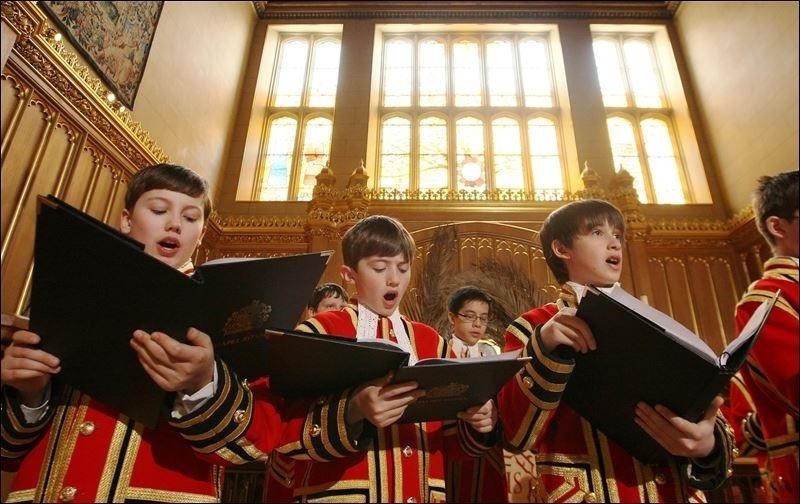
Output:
[498,197,740,504]
[259,213,501,504]
[442,283,507,503]
[0,162,281,504]
[732,169,799,503]
[307,281,351,316]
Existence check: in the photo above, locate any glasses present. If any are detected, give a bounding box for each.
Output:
[455,312,489,323]
